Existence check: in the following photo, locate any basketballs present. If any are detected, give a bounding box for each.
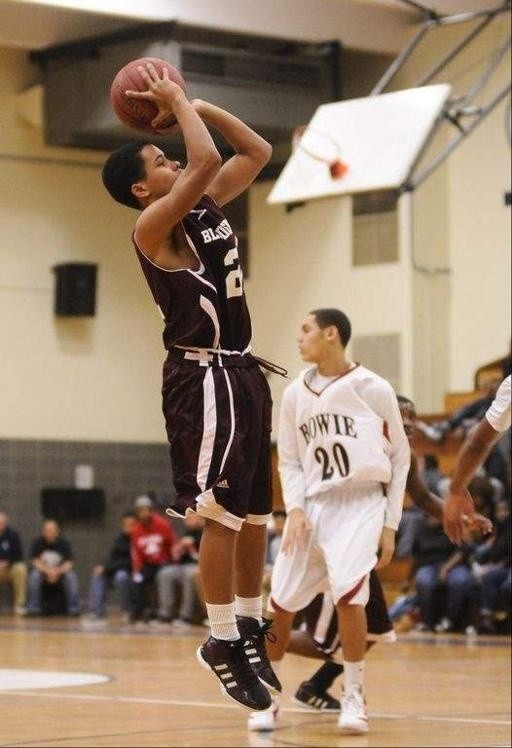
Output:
[112,58,188,134]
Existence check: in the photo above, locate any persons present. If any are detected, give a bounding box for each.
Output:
[245,306,415,735]
[1,510,28,616]
[100,61,291,714]
[27,518,82,615]
[375,355,511,636]
[86,493,206,630]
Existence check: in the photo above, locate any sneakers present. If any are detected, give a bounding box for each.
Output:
[290,680,341,710]
[196,613,282,729]
[337,682,370,732]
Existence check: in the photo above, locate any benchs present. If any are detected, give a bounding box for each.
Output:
[375,360,512,632]
